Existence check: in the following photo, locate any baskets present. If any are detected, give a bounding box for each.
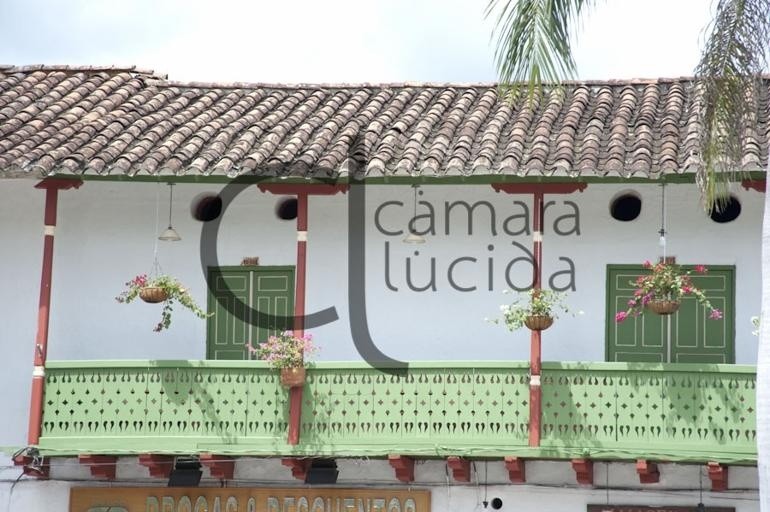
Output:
[647,300,680,314]
[139,286,170,303]
[524,316,553,330]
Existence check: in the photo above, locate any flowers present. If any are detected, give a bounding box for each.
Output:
[488,285,584,331]
[111,274,214,332]
[613,260,722,324]
[247,327,317,369]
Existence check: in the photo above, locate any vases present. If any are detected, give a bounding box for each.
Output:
[526,314,552,330]
[648,298,679,314]
[138,287,167,304]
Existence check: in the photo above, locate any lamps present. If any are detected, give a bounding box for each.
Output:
[163,462,205,485]
[305,459,340,485]
[403,183,424,244]
[655,182,670,248]
[158,182,182,242]
